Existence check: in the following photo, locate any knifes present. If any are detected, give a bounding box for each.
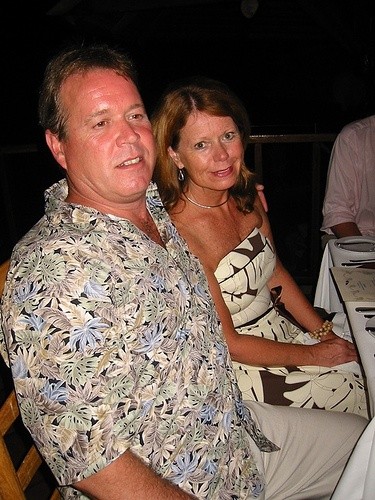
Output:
[336,241,375,245]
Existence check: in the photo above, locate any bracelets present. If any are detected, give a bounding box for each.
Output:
[310,320,333,342]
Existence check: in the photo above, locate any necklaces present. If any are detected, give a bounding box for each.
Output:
[182,189,229,209]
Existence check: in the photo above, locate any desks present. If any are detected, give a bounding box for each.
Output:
[313,239,375,420]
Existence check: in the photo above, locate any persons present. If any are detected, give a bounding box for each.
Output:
[151,86,370,421]
[319,114,375,239]
[0,45,370,500]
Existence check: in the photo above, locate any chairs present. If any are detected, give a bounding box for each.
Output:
[0,259,65,500]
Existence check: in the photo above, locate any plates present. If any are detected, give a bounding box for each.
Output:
[334,236,375,254]
[365,316,375,338]
